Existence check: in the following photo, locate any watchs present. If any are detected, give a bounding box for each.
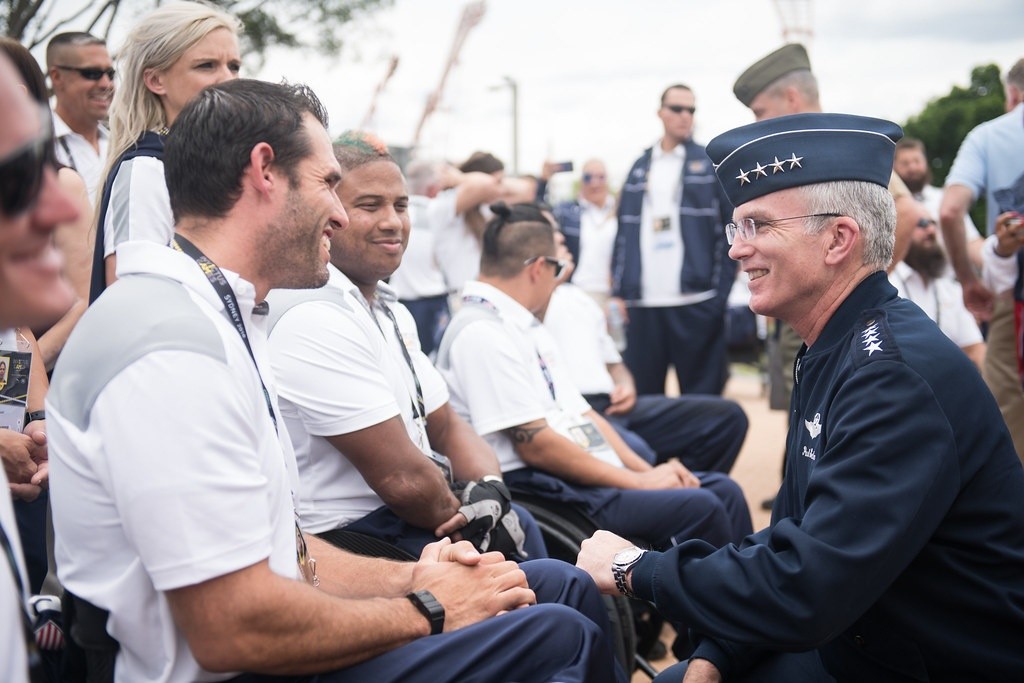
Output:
[612,547,649,601]
[404,589,445,637]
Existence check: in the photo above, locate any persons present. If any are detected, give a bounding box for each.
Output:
[609,84,741,401]
[428,200,755,661]
[43,79,627,683]
[574,162,619,321]
[333,132,571,358]
[889,61,1024,465]
[252,130,548,566]
[90,1,240,306]
[533,205,750,483]
[0,38,115,683]
[731,42,916,512]
[576,113,1024,683]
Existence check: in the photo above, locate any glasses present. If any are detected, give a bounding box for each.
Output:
[725,213,842,245]
[53,65,116,80]
[918,219,935,227]
[523,256,568,279]
[665,104,695,115]
[0,137,58,215]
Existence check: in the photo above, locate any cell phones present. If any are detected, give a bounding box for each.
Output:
[561,161,573,172]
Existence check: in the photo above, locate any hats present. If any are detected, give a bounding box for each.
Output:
[705,113,905,207]
[733,43,811,107]
